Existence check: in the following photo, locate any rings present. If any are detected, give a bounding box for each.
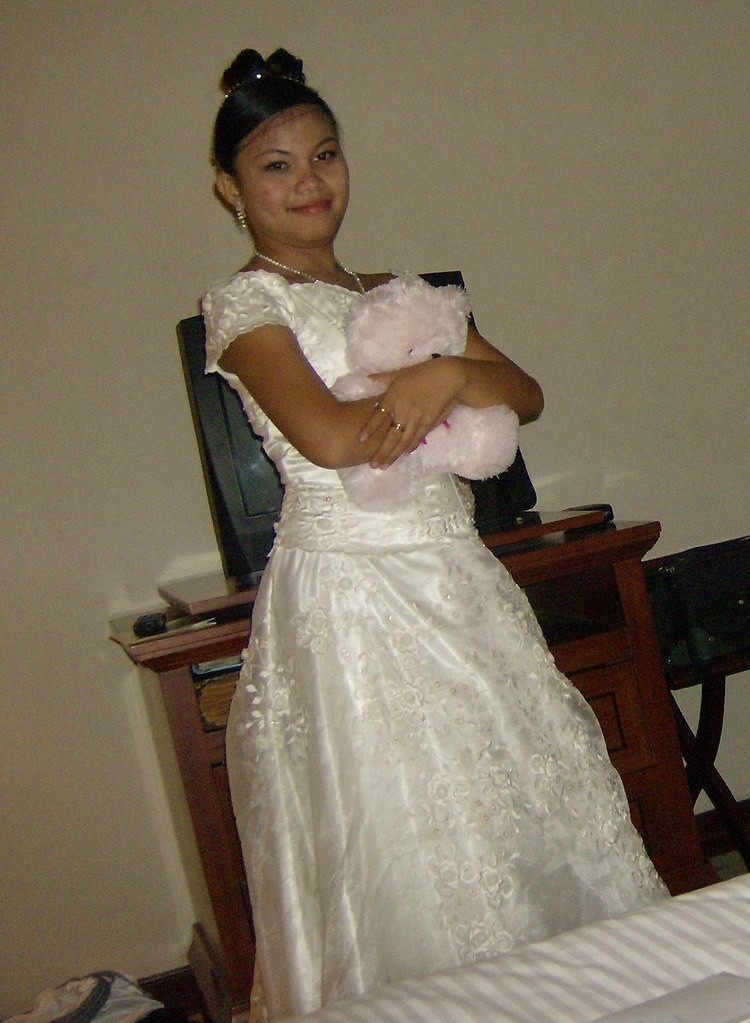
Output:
[391,421,404,431]
[374,401,388,414]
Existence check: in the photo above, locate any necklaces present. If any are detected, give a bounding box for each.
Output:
[254,245,367,295]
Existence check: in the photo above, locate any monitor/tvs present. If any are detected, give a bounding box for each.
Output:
[176,271,543,585]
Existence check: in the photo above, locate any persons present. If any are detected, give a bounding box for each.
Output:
[202,47,672,1023]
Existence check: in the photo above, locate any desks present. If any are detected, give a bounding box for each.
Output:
[103,499,715,1023]
